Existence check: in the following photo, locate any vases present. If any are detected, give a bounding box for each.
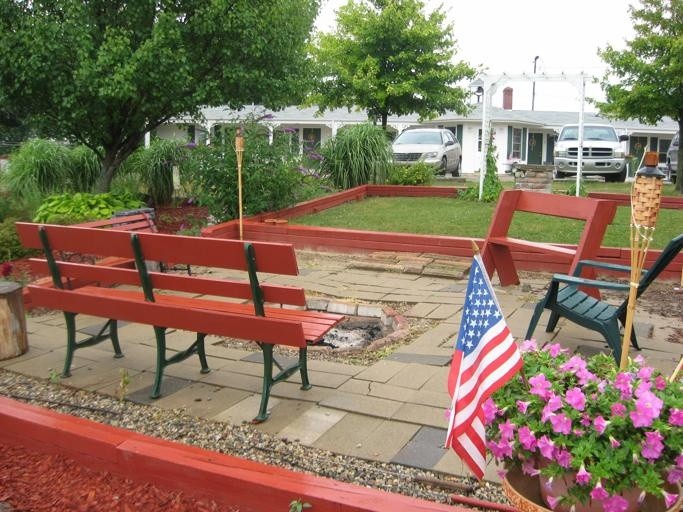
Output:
[442,336,683,512]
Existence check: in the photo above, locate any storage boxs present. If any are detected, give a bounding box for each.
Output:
[14,220,346,424]
[21,211,192,312]
[478,187,618,302]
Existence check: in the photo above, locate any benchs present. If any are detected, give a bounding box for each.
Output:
[444,253,525,482]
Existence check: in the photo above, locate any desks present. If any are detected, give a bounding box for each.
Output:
[530,54,540,109]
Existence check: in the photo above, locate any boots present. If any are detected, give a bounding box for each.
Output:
[553,124,630,183]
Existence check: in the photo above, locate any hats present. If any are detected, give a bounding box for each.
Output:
[665,131,680,180]
[385,127,463,177]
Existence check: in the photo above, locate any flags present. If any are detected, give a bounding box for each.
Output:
[536,462,647,512]
[501,468,683,512]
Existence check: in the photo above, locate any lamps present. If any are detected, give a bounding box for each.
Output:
[524,233,683,370]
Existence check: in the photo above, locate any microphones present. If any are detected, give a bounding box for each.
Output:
[0,280,29,361]
